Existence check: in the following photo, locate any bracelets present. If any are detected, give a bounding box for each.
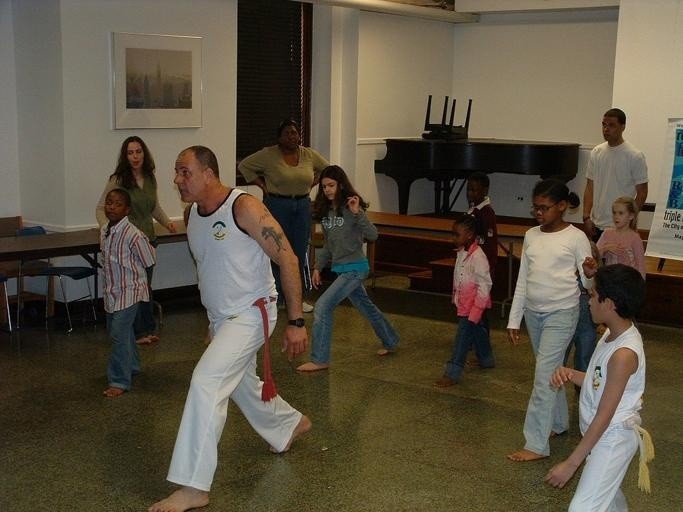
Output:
[285,317,307,328]
[166,222,172,226]
[584,216,590,221]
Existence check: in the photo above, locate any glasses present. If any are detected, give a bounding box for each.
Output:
[530,203,556,212]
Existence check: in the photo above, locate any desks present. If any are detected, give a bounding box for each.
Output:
[0,220,186,261]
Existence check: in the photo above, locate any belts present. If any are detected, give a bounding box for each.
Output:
[270,192,308,199]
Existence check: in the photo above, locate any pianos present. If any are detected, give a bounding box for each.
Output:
[375,136,581,217]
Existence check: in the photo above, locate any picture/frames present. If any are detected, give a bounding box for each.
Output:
[111,31,203,130]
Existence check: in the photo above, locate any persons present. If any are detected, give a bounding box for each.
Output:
[238,121,329,312]
[466,172,497,272]
[96,188,156,398]
[100,135,178,348]
[597,197,647,280]
[540,264,658,511]
[297,165,401,371]
[583,109,649,242]
[504,178,602,463]
[146,144,310,512]
[563,242,600,393]
[433,216,494,387]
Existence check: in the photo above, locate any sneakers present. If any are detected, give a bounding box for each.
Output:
[301,301,313,313]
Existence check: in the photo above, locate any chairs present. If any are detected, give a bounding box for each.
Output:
[422,95,472,141]
[0,216,54,322]
[16,226,97,333]
